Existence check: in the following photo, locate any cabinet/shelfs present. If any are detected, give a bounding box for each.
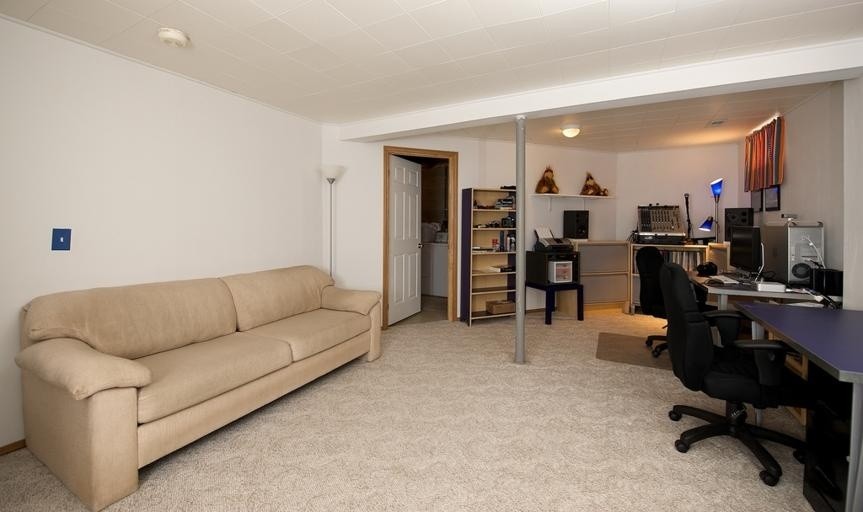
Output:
[524,249,584,326]
[764,323,807,426]
[629,242,727,315]
[422,242,448,299]
[459,185,519,327]
[421,162,448,220]
[555,240,630,316]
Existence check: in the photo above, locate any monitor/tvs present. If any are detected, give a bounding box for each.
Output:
[730,226,762,269]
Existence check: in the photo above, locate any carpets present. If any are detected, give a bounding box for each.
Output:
[10,263,383,510]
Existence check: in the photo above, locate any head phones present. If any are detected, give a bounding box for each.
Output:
[697,261,718,275]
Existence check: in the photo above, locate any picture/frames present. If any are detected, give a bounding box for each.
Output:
[763,183,781,212]
[749,187,763,213]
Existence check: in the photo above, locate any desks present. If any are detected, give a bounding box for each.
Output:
[687,273,841,428]
[731,300,862,512]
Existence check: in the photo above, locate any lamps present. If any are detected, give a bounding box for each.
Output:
[155,26,191,48]
[559,124,581,138]
[697,177,723,242]
[317,162,346,279]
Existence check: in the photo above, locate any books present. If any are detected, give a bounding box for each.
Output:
[472,230,516,273]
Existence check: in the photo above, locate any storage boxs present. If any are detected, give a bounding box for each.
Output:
[484,299,515,316]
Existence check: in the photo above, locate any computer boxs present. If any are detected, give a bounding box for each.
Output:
[762,226,825,289]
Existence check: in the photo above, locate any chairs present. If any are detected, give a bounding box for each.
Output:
[657,261,810,487]
[634,246,718,358]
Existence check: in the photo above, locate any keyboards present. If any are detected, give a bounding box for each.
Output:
[709,275,739,284]
